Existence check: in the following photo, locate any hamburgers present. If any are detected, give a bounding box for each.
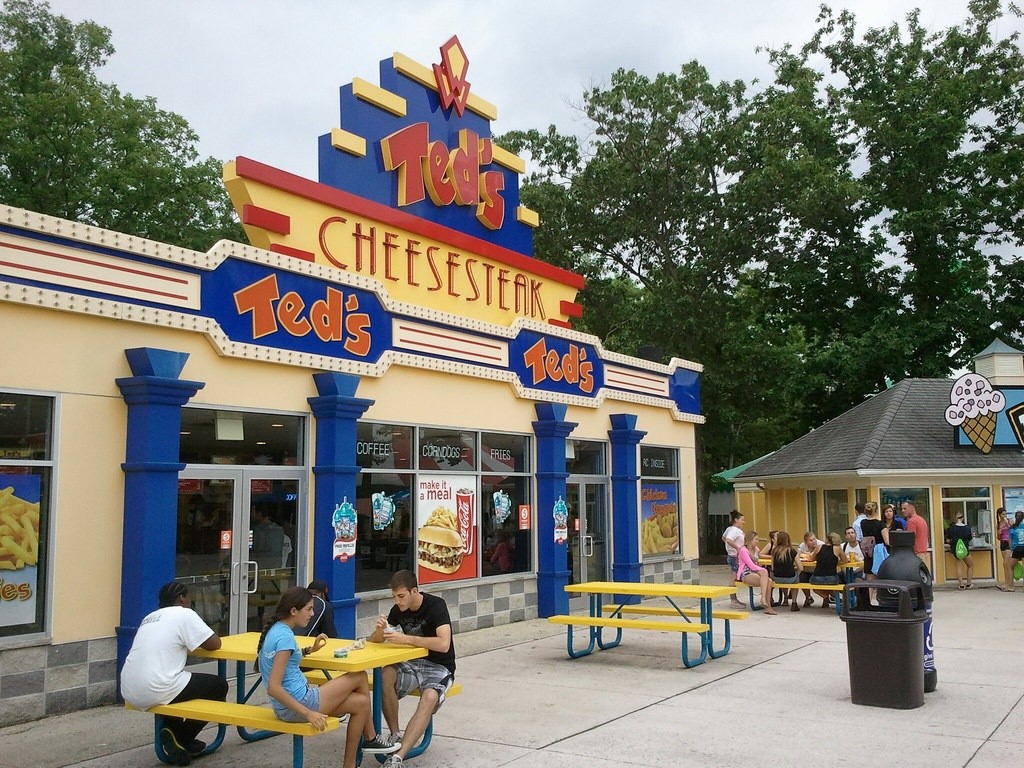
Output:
[418,525,464,573]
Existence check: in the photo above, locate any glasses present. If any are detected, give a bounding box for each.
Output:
[396,507,400,509]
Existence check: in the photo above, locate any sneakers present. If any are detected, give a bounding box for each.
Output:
[361,734,402,753]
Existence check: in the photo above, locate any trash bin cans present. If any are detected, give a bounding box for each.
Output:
[840,578,930,709]
[874,531,939,692]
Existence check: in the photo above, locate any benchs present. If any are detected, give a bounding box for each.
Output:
[602,604,748,658]
[301,669,462,763]
[548,614,710,667]
[734,581,852,615]
[124,700,339,768]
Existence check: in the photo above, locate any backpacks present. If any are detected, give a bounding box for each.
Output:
[1012,563,1022,580]
[955,538,969,561]
[859,519,877,559]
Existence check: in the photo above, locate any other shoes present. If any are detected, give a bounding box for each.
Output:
[764,610,777,614]
[996,584,1014,592]
[760,601,768,606]
[787,591,792,599]
[821,597,829,607]
[829,597,842,604]
[803,597,814,607]
[161,723,191,766]
[966,583,974,589]
[174,734,206,754]
[791,603,800,611]
[379,756,403,768]
[385,732,405,758]
[783,598,788,606]
[338,714,346,722]
[730,598,746,609]
[958,585,966,590]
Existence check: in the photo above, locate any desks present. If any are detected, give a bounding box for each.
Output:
[756,558,862,585]
[186,632,428,741]
[564,582,738,624]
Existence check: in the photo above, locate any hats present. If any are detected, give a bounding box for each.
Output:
[397,501,403,507]
[308,582,330,602]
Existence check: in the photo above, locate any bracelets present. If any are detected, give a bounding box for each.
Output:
[301,647,310,657]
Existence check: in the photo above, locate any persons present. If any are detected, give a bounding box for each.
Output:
[946,512,975,590]
[1001,511,1024,592]
[995,507,1015,590]
[119,581,222,767]
[290,580,347,724]
[253,587,402,768]
[249,500,284,591]
[722,500,929,615]
[370,570,456,768]
[489,533,515,573]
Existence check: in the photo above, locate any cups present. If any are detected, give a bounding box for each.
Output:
[849,552,856,563]
[383,627,396,642]
[456,492,474,555]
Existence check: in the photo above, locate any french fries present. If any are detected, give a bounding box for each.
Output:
[0,486,40,570]
[427,507,459,531]
[642,519,662,554]
